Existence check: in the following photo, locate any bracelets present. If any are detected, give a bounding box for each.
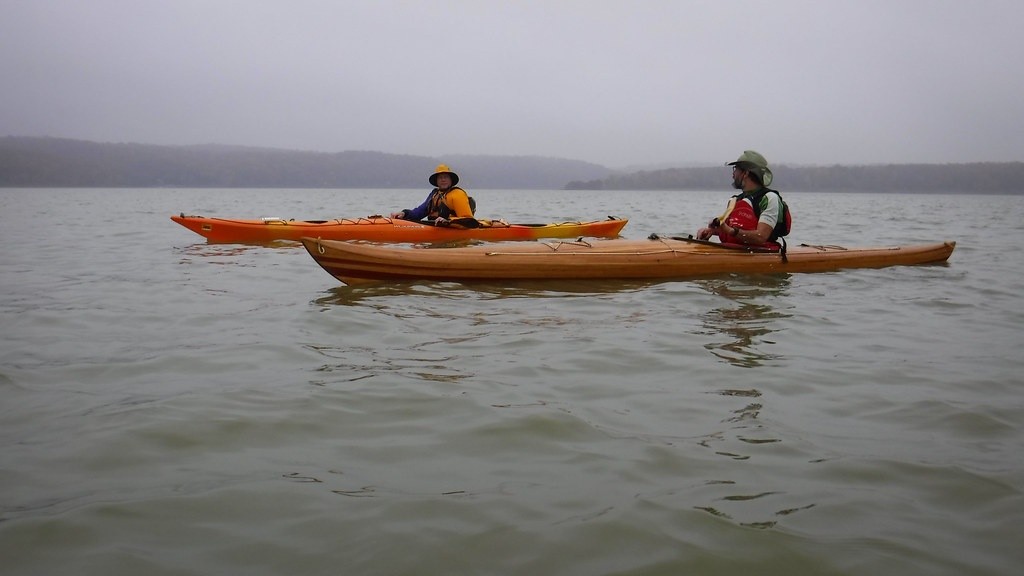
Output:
[732,226,739,237]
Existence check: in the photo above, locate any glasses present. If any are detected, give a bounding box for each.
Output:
[733,165,741,172]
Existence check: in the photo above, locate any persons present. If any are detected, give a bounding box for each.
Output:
[390,164,474,226]
[696,150,791,251]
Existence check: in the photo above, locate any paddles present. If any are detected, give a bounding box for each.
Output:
[705,197,737,241]
[421,217,479,227]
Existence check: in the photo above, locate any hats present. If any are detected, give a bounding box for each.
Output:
[727,151,773,186]
[428,164,459,187]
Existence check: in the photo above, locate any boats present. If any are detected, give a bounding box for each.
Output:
[299,233,957,285]
[170,212,631,246]
